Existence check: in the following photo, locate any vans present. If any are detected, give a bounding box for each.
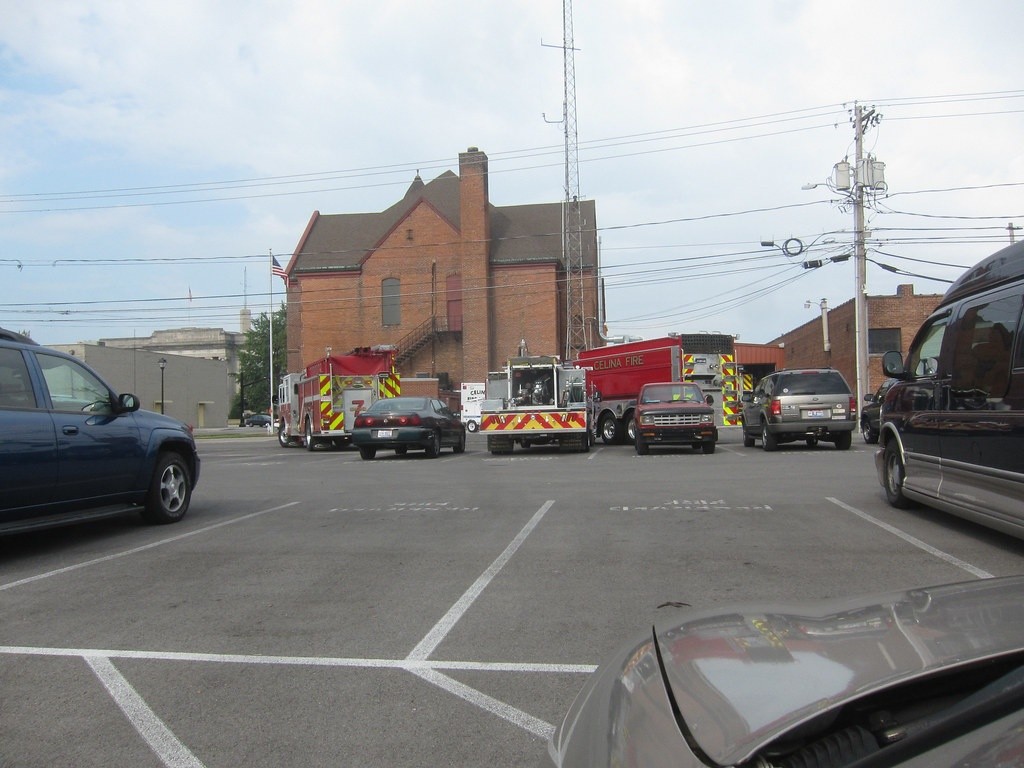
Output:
[875,242,1024,544]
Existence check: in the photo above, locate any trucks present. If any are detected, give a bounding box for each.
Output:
[459,383,487,432]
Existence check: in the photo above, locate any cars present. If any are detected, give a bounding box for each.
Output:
[246,413,276,428]
[545,573,1024,768]
[351,397,467,458]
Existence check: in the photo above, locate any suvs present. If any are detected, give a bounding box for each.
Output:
[741,368,857,453]
[627,382,717,454]
[0,337,201,539]
[859,378,902,443]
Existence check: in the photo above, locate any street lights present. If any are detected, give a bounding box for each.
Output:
[801,181,868,440]
[156,357,168,416]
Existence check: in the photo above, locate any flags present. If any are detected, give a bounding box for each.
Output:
[271,254,289,289]
[188,288,193,302]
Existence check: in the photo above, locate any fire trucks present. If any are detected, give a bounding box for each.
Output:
[272,344,402,452]
[480,337,596,453]
[564,331,743,446]
